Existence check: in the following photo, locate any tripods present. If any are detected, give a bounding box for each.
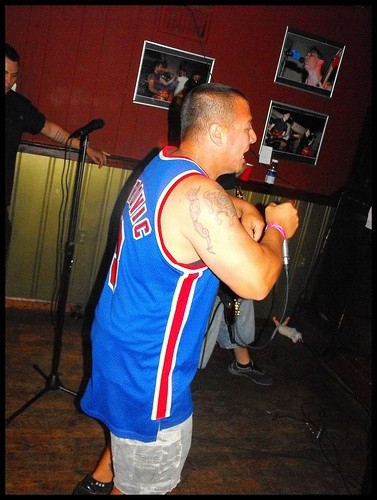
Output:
[5,135,89,426]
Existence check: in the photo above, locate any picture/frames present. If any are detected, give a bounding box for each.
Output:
[132,39,216,110]
[273,25,347,99]
[258,100,329,168]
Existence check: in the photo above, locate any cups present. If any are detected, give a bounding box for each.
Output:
[239,164,255,182]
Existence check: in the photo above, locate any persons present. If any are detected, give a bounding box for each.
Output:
[264,112,319,158]
[5,41,111,266]
[71,84,300,498]
[303,44,336,91]
[144,60,205,104]
[215,299,274,386]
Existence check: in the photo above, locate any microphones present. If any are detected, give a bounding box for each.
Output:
[282,239,289,270]
[71,119,104,138]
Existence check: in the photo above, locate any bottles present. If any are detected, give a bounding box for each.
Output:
[263,158,280,188]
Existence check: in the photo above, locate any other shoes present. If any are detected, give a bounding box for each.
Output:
[228,358,273,385]
[72,478,99,495]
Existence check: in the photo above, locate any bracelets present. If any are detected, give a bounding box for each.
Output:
[266,223,286,239]
